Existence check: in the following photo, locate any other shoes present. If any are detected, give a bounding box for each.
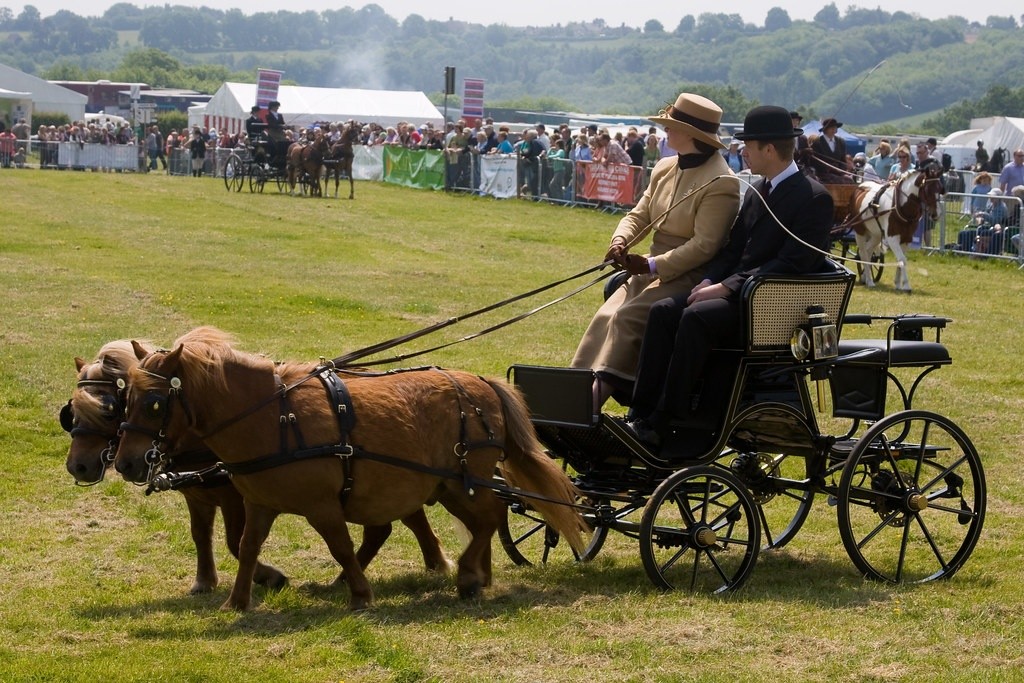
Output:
[615,415,665,448]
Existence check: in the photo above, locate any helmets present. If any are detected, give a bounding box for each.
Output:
[976,139,984,146]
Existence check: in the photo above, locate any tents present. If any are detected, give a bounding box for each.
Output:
[187,82,445,143]
[940,129,982,145]
[800,120,866,155]
[966,117,1024,165]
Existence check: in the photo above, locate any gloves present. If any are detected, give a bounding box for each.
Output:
[600,244,650,275]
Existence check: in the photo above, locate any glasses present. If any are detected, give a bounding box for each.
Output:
[897,155,907,159]
[659,103,674,119]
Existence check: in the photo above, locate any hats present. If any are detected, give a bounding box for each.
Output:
[585,123,598,132]
[269,101,280,110]
[733,105,805,141]
[534,123,546,130]
[76,119,84,125]
[987,187,1003,195]
[419,124,429,130]
[790,110,803,122]
[250,106,260,114]
[219,127,228,131]
[818,118,843,133]
[648,93,731,149]
[190,123,200,128]
[103,122,115,130]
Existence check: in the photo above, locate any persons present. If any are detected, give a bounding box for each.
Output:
[0,118,249,178]
[788,112,820,181]
[285,118,678,216]
[191,131,206,177]
[610,106,837,453]
[811,120,847,182]
[265,101,288,142]
[246,105,269,157]
[570,92,742,422]
[847,136,1024,259]
[722,141,744,174]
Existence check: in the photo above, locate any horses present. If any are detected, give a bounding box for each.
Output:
[847,158,946,293]
[287,121,361,199]
[68,327,595,617]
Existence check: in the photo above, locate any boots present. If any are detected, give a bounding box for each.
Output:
[193,169,201,177]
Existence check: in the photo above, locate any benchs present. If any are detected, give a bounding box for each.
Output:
[248,123,295,153]
[603,260,861,352]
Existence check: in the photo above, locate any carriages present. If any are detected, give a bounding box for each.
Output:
[61,261,986,617]
[814,162,949,297]
[224,121,364,200]
